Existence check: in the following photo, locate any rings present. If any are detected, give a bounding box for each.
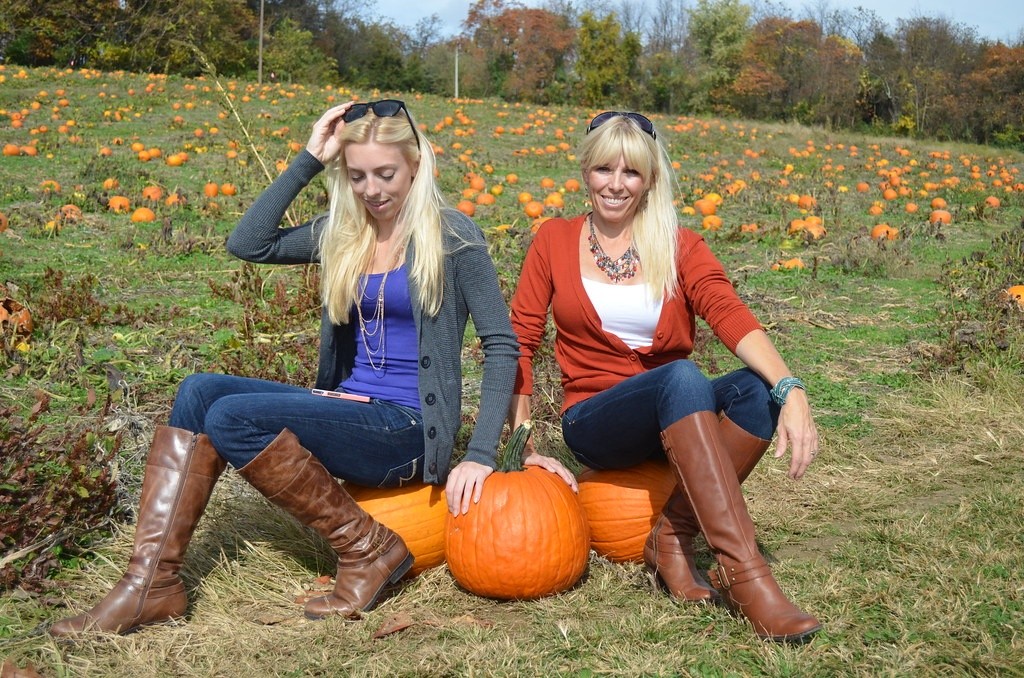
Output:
[811,450,816,455]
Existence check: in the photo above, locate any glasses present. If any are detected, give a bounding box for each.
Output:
[340,100,420,150]
[588,112,657,141]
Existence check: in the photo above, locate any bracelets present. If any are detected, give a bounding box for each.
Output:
[771,377,809,404]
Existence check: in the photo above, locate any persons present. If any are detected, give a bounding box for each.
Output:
[47,100,520,640]
[509,113,824,645]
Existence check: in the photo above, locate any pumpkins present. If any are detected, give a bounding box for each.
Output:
[337,479,453,578]
[0,63,1024,337]
[444,420,591,601]
[572,458,674,565]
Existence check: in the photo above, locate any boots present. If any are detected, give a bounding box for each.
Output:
[53,426,227,641]
[661,410,820,640]
[644,410,773,608]
[236,425,416,622]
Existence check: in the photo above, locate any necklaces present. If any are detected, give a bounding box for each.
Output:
[587,212,640,280]
[353,255,395,371]
